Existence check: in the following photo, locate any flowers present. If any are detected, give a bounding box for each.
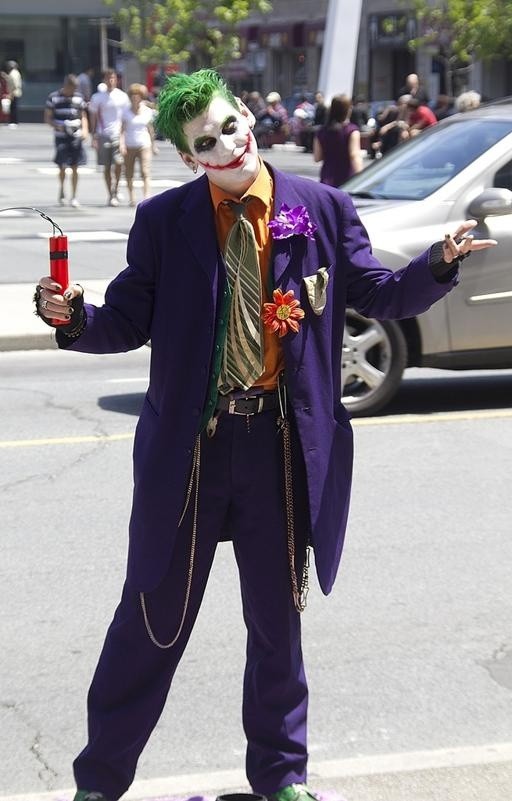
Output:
[262,289,306,339]
[267,202,318,245]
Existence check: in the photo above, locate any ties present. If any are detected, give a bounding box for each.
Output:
[216,199,265,395]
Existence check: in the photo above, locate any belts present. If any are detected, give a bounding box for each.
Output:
[216,391,281,415]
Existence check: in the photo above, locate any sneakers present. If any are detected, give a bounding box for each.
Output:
[59,194,137,208]
[253,781,323,800]
[72,790,106,800]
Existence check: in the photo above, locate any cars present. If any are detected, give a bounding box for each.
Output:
[328,97,511,418]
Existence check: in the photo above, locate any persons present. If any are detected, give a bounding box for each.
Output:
[34,66,499,800]
[241,73,481,189]
[44,69,177,206]
[0,59,23,125]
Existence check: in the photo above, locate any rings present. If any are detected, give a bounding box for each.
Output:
[454,253,460,258]
[41,300,48,307]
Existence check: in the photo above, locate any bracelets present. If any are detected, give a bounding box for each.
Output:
[62,317,86,339]
